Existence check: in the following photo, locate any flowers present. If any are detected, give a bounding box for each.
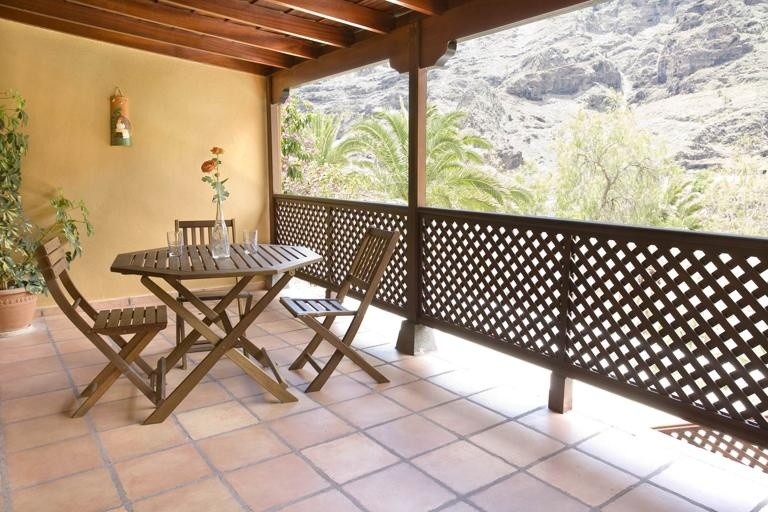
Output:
[201,145,231,252]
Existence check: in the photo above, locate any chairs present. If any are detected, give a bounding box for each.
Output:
[34,218,401,419]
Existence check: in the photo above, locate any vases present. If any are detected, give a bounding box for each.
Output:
[211,203,230,259]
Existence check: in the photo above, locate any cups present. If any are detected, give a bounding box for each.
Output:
[243,230,258,254]
[167,230,183,257]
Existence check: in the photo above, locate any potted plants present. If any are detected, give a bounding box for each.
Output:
[1,89,94,333]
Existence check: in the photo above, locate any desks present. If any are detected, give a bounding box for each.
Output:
[110,243,324,425]
